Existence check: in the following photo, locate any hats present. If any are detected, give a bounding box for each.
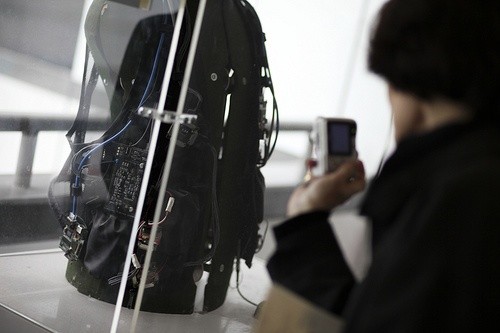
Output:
[364,0,500,111]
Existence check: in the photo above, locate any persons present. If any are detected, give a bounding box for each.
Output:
[253,0,500,333]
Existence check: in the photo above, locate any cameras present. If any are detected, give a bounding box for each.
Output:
[312,117,357,181]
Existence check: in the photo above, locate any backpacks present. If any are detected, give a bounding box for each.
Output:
[48,3,281,315]
